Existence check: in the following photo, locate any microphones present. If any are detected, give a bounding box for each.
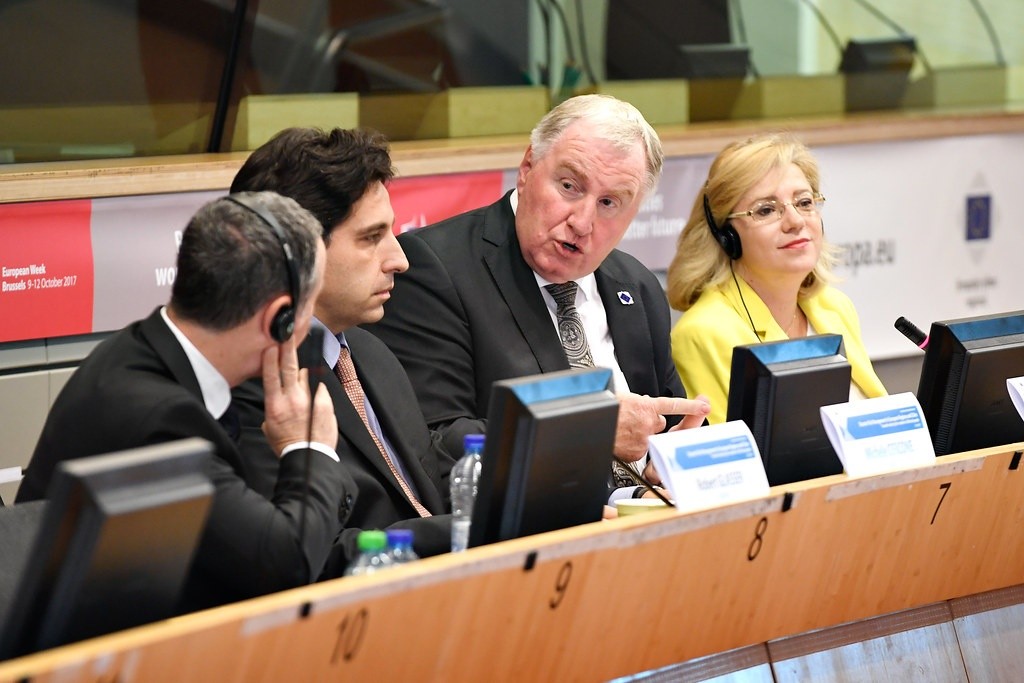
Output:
[894,316,929,351]
[295,325,326,584]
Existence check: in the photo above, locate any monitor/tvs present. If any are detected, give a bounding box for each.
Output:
[727,334,852,488]
[659,45,750,123]
[467,368,619,549]
[838,37,920,113]
[915,310,1024,457]
[0,438,216,665]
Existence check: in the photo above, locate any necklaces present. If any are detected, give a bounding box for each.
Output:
[785,308,799,336]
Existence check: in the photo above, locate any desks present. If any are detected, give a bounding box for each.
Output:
[1,442,1024,683]
[0,106,1023,343]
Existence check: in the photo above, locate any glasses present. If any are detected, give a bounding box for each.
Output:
[728,193,825,224]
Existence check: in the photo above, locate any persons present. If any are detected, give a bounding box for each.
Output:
[14,189,359,621]
[357,92,711,487]
[667,132,891,426]
[229,125,671,561]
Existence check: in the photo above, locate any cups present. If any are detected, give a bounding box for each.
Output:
[614,498,675,518]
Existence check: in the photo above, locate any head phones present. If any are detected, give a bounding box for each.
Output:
[703,183,742,261]
[223,194,300,342]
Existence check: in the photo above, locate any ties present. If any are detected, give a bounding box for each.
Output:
[543,280,643,489]
[334,347,433,519]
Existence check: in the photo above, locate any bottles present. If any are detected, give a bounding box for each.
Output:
[450,434,486,552]
[348,529,393,574]
[385,528,418,563]
